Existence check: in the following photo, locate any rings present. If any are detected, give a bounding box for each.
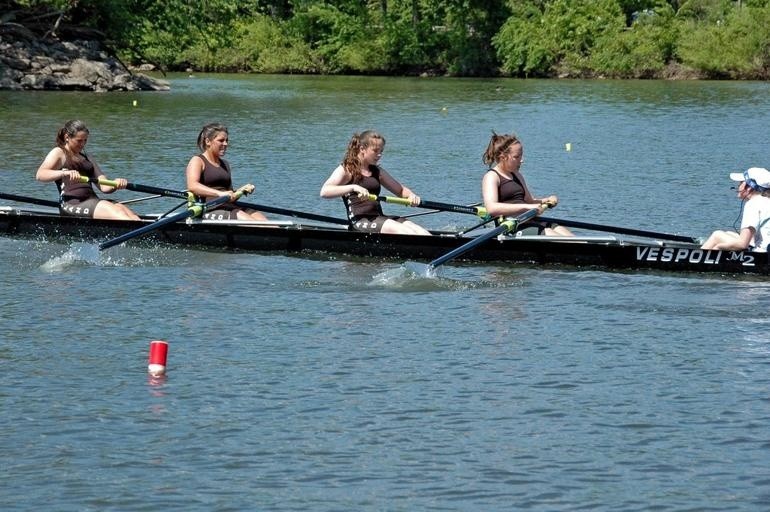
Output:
[73,171,75,173]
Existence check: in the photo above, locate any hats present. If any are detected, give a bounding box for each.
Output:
[728,166,770,190]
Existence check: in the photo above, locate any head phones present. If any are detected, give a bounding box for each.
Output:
[744,170,756,193]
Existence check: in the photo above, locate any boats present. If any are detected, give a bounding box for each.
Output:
[0,206,770,276]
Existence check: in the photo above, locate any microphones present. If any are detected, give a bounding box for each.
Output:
[730,185,748,193]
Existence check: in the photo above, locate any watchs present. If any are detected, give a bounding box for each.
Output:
[60,168,69,179]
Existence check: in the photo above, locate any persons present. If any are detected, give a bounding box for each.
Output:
[185,123,268,221]
[35,119,143,221]
[700,166,770,253]
[318,129,434,235]
[480,131,577,237]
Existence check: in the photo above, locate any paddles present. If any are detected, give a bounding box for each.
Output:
[403,200,552,279]
[71,190,248,261]
